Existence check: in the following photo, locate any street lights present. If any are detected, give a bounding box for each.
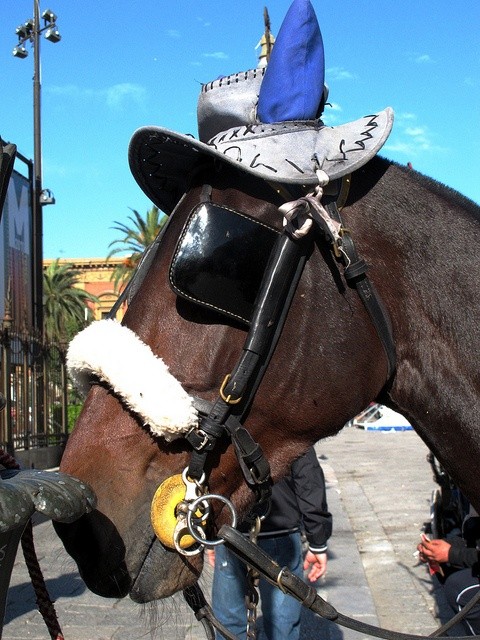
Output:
[12,10,61,447]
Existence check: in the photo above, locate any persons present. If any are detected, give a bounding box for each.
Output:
[413,509,480,637]
[207,445,335,640]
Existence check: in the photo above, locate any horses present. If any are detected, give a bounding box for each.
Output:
[52,0,480,607]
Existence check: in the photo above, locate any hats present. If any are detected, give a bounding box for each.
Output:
[126,2,394,216]
